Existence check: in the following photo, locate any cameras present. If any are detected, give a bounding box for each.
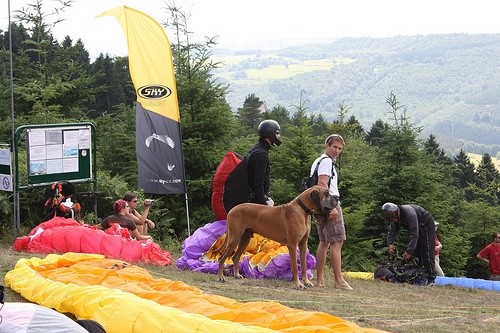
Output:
[144,199,155,206]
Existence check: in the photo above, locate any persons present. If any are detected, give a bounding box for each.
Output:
[222,120,282,214]
[310,134,355,291]
[381,202,435,277]
[434,220,445,276]
[77,192,155,240]
[476,233,500,281]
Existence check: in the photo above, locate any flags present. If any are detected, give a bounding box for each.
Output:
[95,7,188,193]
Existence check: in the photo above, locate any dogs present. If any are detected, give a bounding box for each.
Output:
[209,185,341,292]
